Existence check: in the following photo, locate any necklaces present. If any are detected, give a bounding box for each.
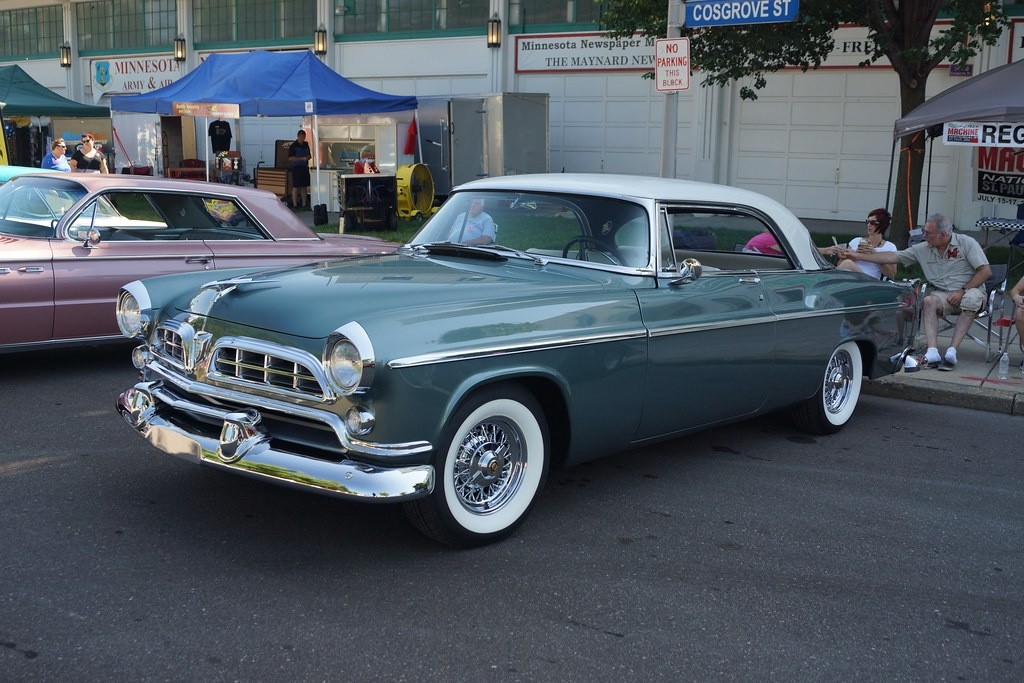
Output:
[875,239,883,248]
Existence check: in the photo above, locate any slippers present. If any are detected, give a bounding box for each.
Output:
[922,357,955,371]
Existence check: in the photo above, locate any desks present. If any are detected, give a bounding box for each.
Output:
[166,167,215,183]
[975,217,1024,252]
[213,169,241,188]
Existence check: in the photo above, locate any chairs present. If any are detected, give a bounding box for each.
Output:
[180,158,205,181]
[735,244,1024,362]
[1007,202,1024,279]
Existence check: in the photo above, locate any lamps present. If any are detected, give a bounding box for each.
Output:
[59,41,71,67]
[486,13,501,47]
[174,34,186,61]
[314,24,327,54]
[984,2,997,26]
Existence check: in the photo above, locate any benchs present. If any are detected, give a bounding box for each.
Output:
[619,245,791,273]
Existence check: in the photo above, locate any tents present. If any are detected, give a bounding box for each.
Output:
[0,64,113,133]
[110,51,423,207]
[885,58,1024,223]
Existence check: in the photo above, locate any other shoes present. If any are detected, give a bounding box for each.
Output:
[1020,360,1024,374]
[904,355,919,373]
[294,205,308,212]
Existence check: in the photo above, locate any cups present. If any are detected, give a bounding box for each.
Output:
[838,244,847,253]
[859,244,867,253]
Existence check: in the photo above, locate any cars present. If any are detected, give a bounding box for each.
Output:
[116,173,920,545]
[0,169,390,359]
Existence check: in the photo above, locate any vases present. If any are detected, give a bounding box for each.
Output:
[234,157,238,170]
[216,157,219,168]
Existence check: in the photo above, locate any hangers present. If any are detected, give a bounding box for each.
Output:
[216,117,225,123]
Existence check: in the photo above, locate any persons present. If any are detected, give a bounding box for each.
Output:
[288,130,312,212]
[445,199,496,247]
[743,227,843,257]
[1010,275,1024,370]
[354,145,380,174]
[69,134,110,175]
[838,213,992,364]
[41,138,72,173]
[836,208,897,281]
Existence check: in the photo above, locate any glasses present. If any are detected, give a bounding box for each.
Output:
[922,229,941,236]
[82,138,91,143]
[56,144,66,149]
[865,218,879,224]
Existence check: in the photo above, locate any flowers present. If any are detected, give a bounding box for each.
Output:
[217,151,228,158]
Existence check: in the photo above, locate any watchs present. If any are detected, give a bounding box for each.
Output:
[961,287,969,293]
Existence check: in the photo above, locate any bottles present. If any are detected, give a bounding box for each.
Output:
[997,353,1010,380]
